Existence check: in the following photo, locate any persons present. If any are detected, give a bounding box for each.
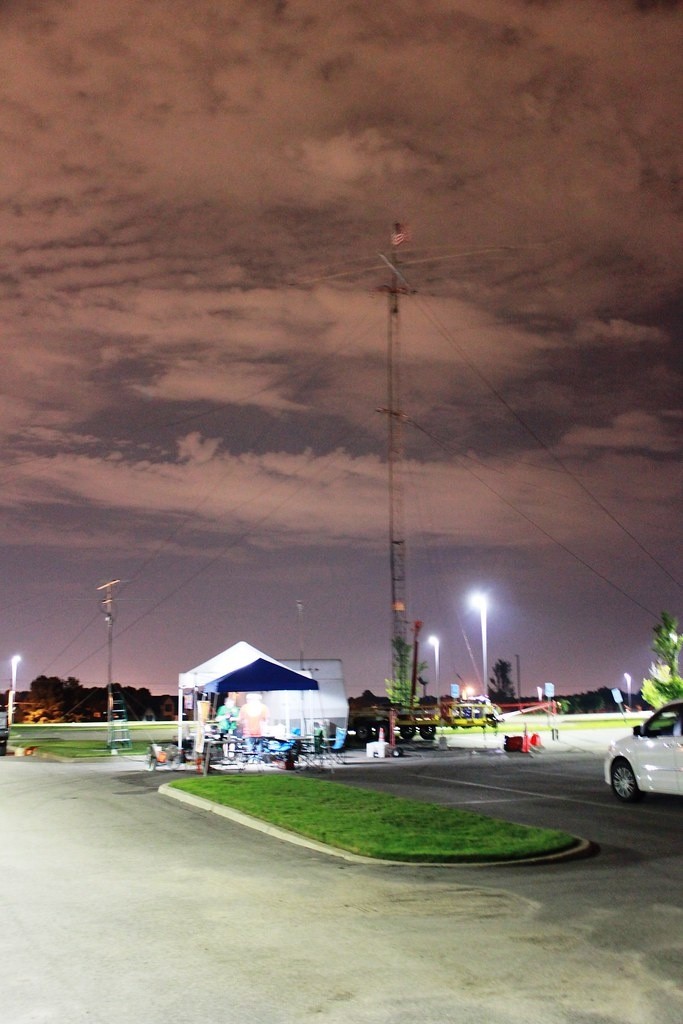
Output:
[237,694,268,764]
[215,697,239,761]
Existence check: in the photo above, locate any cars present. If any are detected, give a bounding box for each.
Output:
[603,700,683,805]
[0,711,10,757]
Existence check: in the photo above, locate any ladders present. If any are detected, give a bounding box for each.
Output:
[111,691,133,751]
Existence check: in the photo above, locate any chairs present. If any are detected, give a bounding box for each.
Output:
[318,727,349,766]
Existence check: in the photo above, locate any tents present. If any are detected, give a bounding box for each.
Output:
[178,641,320,754]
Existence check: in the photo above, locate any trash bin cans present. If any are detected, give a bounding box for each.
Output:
[0,728,9,756]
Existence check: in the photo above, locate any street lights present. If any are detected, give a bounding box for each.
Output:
[471,592,489,696]
[12,654,21,696]
[623,673,633,709]
[427,635,440,700]
